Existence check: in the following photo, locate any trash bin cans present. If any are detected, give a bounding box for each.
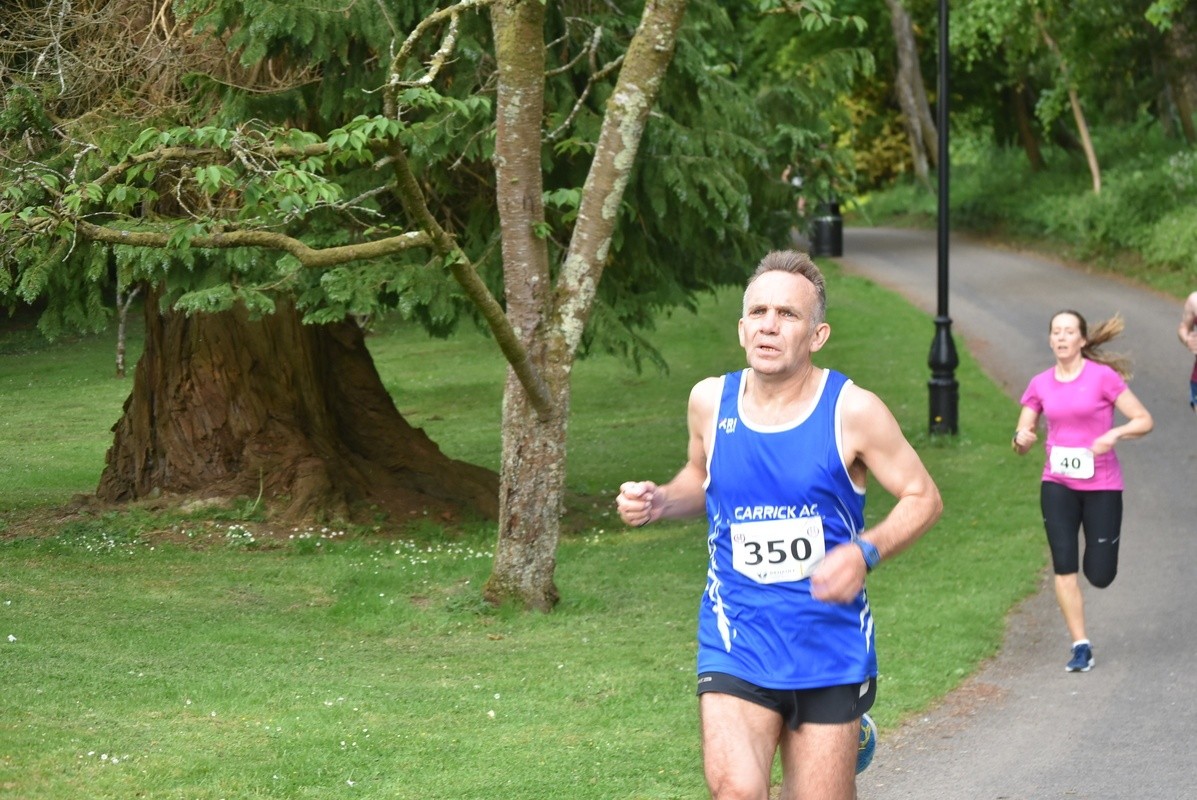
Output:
[812,204,844,258]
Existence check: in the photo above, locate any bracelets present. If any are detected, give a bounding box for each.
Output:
[855,537,881,571]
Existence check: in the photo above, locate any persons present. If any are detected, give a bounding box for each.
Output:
[1013,307,1154,673]
[1176,292,1197,411]
[616,247,944,800]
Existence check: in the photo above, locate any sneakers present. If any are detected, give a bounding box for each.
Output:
[853,709,877,776]
[1065,642,1094,673]
